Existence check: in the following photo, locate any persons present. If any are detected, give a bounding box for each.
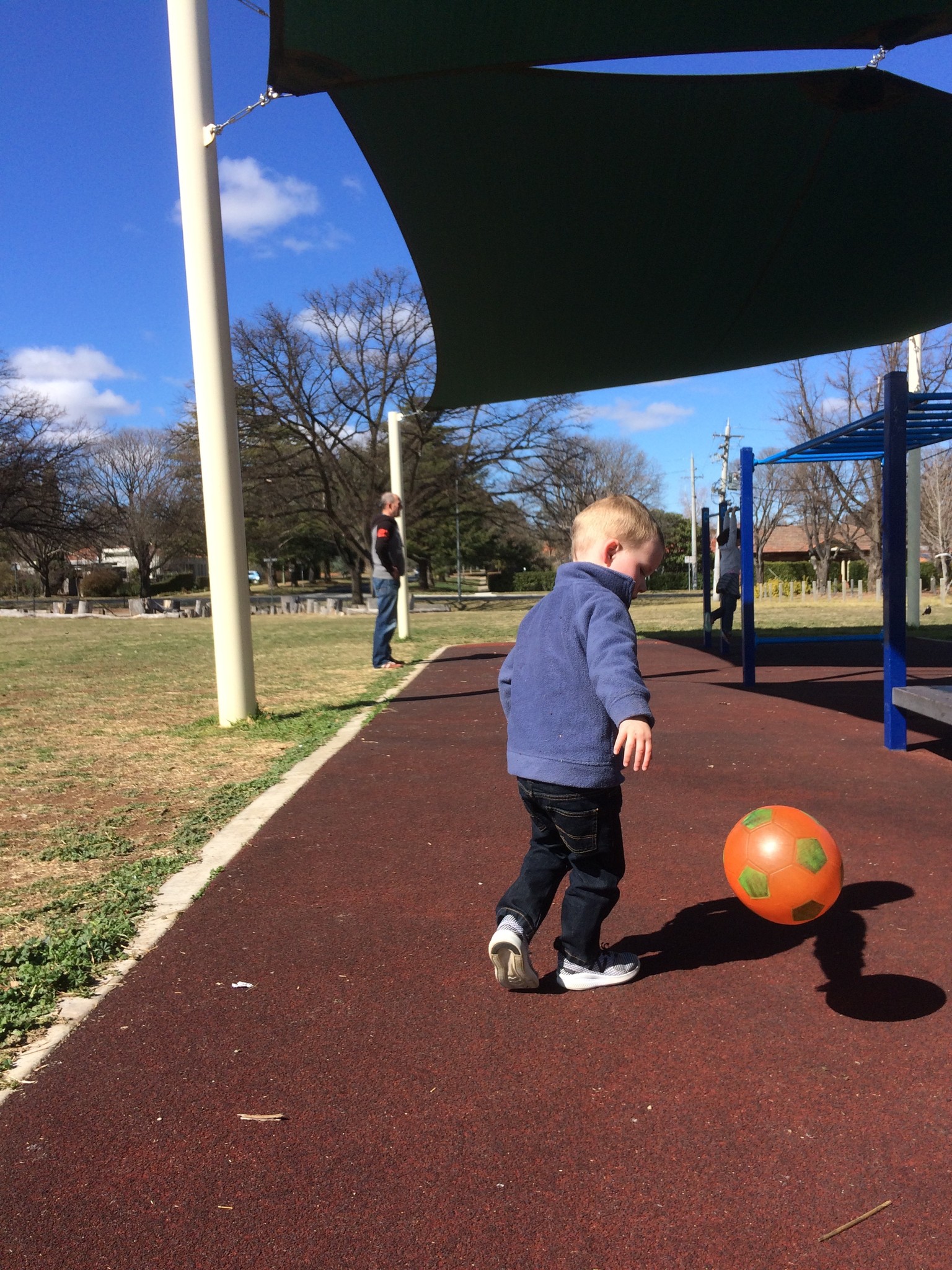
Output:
[704,506,742,644]
[487,496,662,992]
[370,491,405,669]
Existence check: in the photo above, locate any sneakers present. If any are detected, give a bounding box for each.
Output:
[381,657,405,668]
[704,612,714,633]
[556,949,642,990]
[720,631,732,645]
[488,913,540,989]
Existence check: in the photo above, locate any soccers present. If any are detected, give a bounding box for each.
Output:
[722,804,844,926]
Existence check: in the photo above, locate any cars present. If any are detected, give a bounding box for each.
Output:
[248,570,261,585]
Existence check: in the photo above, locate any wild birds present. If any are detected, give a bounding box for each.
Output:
[922,605,931,615]
